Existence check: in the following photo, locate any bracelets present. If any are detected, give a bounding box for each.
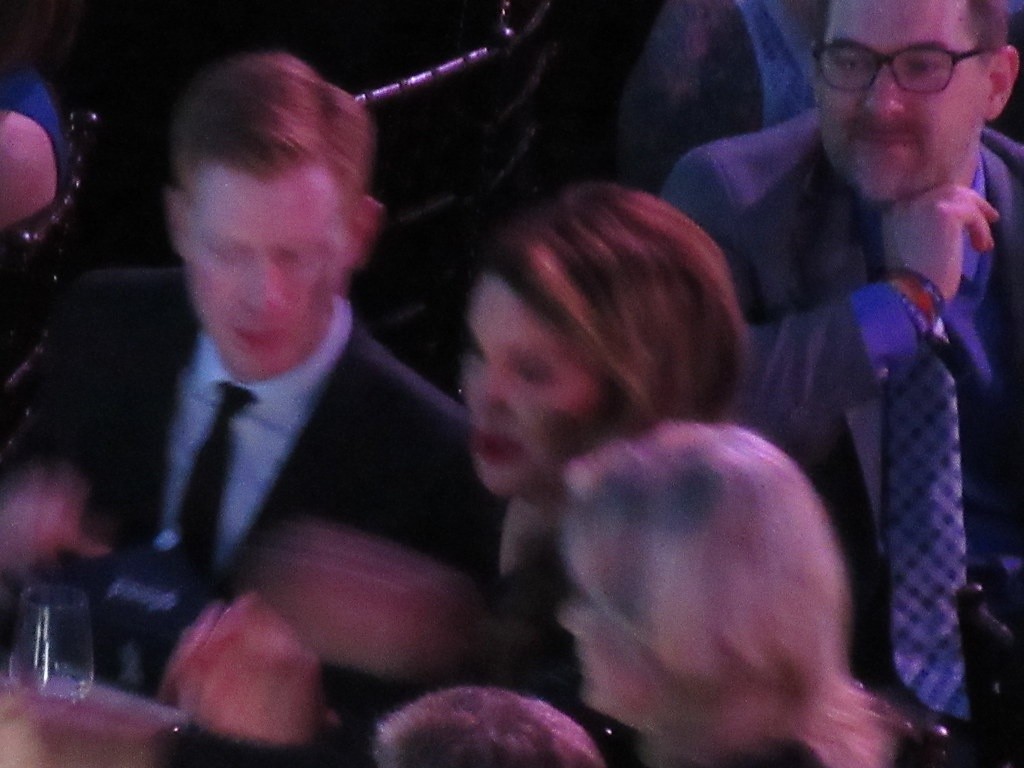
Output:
[892,268,946,334]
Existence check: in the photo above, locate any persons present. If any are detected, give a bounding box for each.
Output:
[0,0,1024,767]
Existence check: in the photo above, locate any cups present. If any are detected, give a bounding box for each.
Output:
[8,586,95,721]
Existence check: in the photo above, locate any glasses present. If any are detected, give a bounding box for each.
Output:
[810,38,985,94]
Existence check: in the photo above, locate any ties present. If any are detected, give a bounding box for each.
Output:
[178,383,259,597]
[879,355,968,719]
